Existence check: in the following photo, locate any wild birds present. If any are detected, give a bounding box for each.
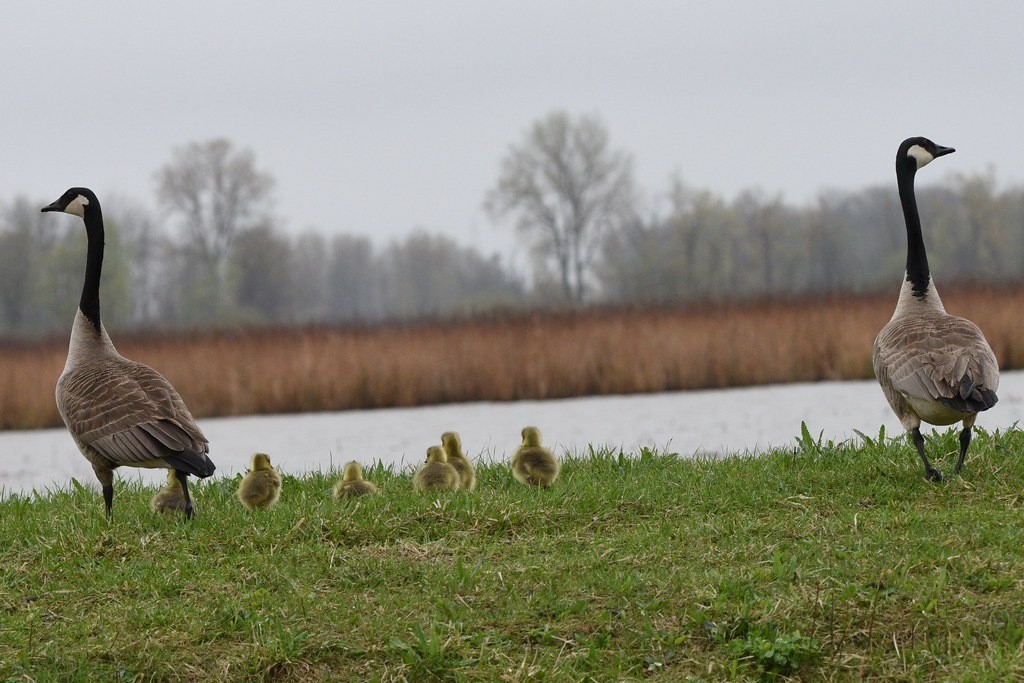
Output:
[152,468,197,517]
[439,431,475,492]
[333,460,377,500]
[511,427,560,489]
[237,452,281,511]
[871,136,1001,486]
[40,187,217,523]
[414,446,459,493]
